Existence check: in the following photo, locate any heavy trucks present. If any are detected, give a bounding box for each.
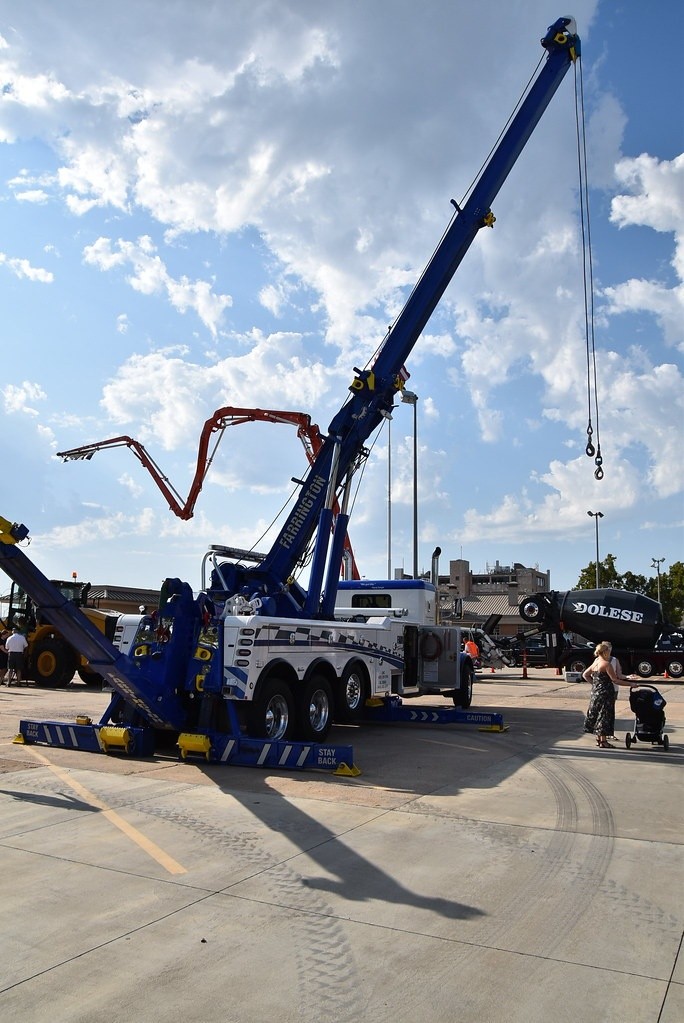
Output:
[102,546,476,743]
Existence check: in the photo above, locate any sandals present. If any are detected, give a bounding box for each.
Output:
[601,740,615,748]
[596,740,601,746]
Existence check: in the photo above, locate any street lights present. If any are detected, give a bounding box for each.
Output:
[652,558,665,604]
[586,511,605,589]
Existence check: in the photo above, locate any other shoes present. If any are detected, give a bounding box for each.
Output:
[16,684,20,687]
[473,679,478,683]
[1,683,6,685]
[8,684,10,687]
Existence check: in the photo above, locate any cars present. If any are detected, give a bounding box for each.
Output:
[454,626,549,669]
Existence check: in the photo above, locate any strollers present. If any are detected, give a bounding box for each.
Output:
[626,685,670,751]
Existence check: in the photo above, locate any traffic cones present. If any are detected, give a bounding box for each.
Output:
[663,669,671,678]
[490,666,496,673]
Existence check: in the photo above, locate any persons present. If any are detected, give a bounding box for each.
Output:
[0,629,9,686]
[463,637,479,663]
[582,645,637,748]
[602,641,622,741]
[5,628,28,688]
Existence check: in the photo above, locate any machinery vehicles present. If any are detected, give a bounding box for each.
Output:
[519,585,684,679]
[0,14,604,778]
[0,572,124,689]
[57,406,362,581]
